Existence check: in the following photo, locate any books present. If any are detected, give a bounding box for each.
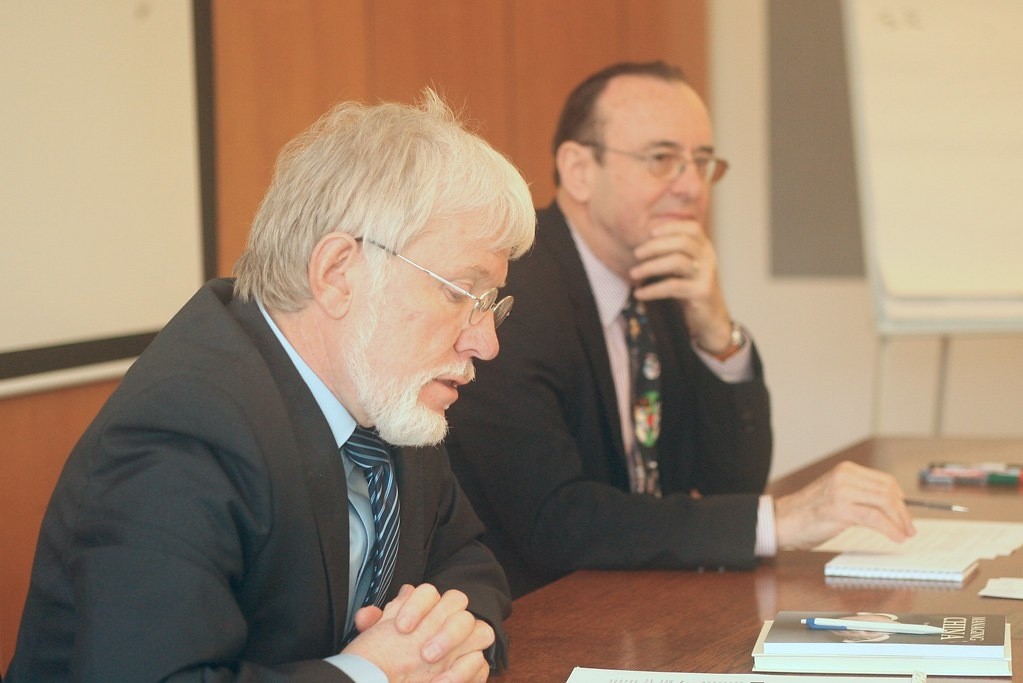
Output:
[751,610,1012,677]
[824,552,980,588]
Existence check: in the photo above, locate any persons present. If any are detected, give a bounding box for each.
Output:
[1,83,537,683]
[445,60,915,601]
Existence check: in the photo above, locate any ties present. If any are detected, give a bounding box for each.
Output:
[622,288,663,496]
[342,426,401,649]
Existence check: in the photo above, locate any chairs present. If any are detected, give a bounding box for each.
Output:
[0,331,162,682]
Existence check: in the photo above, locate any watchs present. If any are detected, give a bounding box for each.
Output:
[697,320,744,361]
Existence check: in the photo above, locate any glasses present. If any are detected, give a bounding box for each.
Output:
[356,237,514,329]
[581,140,728,187]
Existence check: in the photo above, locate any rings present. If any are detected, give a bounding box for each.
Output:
[690,260,700,278]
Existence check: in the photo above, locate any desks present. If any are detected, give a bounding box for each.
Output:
[486,437,1023,683]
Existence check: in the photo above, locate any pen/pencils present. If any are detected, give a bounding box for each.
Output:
[800,617,949,634]
[904,499,970,515]
